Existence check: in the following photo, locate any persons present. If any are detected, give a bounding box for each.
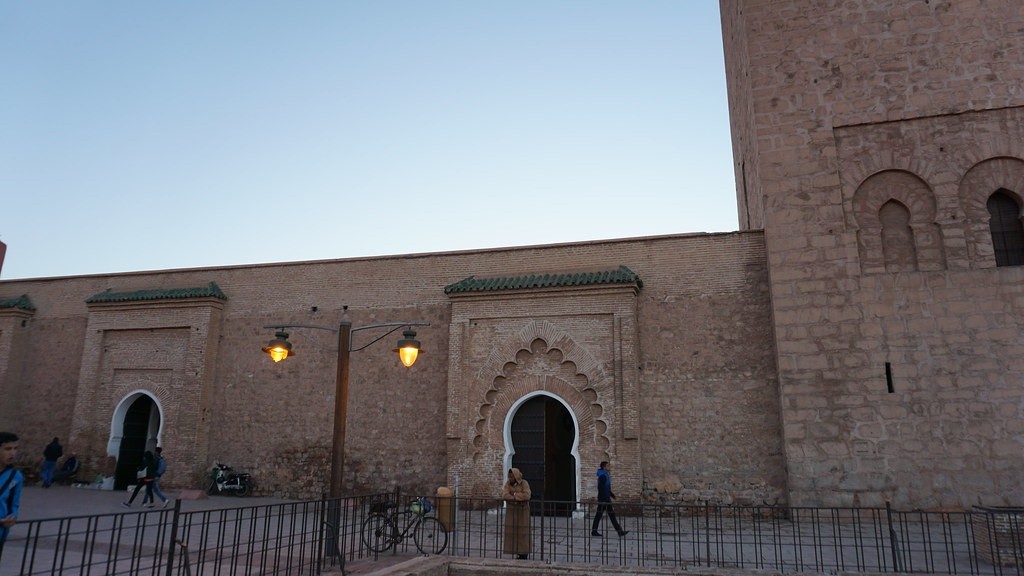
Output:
[42,437,63,487]
[590,461,631,538]
[121,451,156,508]
[141,446,170,508]
[502,468,533,560]
[0,431,24,562]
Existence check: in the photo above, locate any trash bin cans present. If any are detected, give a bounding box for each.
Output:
[435,487,456,533]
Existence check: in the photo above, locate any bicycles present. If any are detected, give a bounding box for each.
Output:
[362,492,449,558]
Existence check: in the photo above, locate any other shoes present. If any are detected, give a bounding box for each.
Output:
[163,499,169,508]
[41,479,50,488]
[148,504,155,509]
[121,502,131,508]
[140,503,147,508]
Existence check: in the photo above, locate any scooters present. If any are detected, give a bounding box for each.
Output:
[207,460,254,499]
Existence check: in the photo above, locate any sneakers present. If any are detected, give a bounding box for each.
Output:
[618,530,629,537]
[592,530,602,536]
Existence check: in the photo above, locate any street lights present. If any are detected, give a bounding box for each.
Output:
[258,304,434,565]
